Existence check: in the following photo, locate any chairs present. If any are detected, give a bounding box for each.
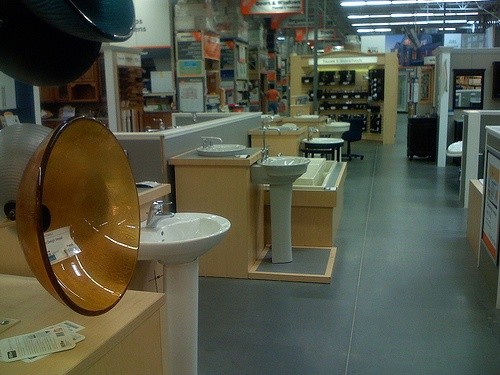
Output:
[342,117,364,160]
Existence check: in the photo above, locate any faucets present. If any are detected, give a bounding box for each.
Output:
[145,111,198,132]
[200,136,223,150]
[267,110,274,116]
[261,129,282,163]
[296,109,303,116]
[307,128,318,141]
[263,115,273,128]
[326,120,335,124]
[142,201,175,232]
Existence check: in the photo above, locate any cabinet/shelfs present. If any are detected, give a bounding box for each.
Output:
[40,59,103,103]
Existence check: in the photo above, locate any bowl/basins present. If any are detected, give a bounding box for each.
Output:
[0,0,103,86]
[16,114,142,317]
[21,0,136,42]
[0,123,53,219]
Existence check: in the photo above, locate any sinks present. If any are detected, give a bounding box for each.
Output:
[0,0,136,93]
[326,122,350,131]
[266,158,340,191]
[262,157,311,176]
[256,126,298,131]
[303,137,344,147]
[294,115,319,119]
[0,116,140,316]
[197,144,246,157]
[261,115,280,119]
[137,212,232,263]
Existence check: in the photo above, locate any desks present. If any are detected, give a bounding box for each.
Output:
[0,274,167,375]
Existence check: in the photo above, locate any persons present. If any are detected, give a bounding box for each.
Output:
[266,83,283,114]
